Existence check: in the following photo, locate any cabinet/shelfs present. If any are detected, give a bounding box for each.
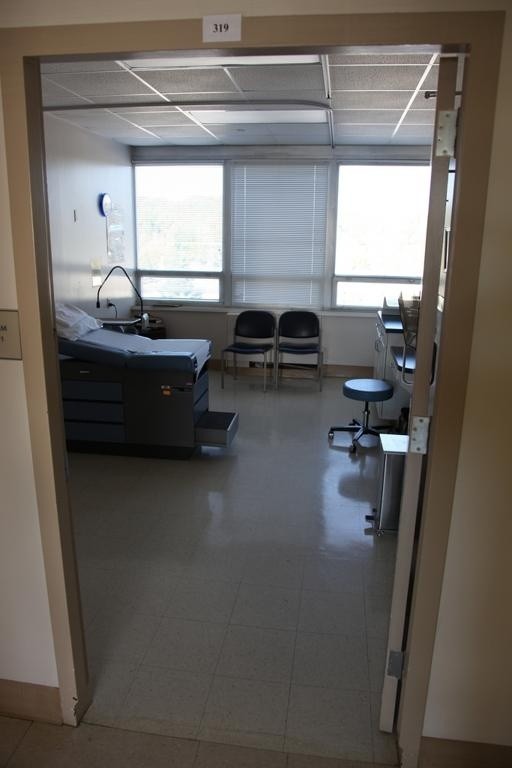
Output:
[372,316,387,420]
[59,359,239,448]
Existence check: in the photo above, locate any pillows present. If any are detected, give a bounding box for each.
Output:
[54,302,103,341]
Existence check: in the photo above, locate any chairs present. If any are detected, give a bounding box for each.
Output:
[219,309,323,392]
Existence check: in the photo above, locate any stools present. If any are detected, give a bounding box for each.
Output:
[328,377,395,454]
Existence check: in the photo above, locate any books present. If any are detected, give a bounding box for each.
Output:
[130,315,166,328]
